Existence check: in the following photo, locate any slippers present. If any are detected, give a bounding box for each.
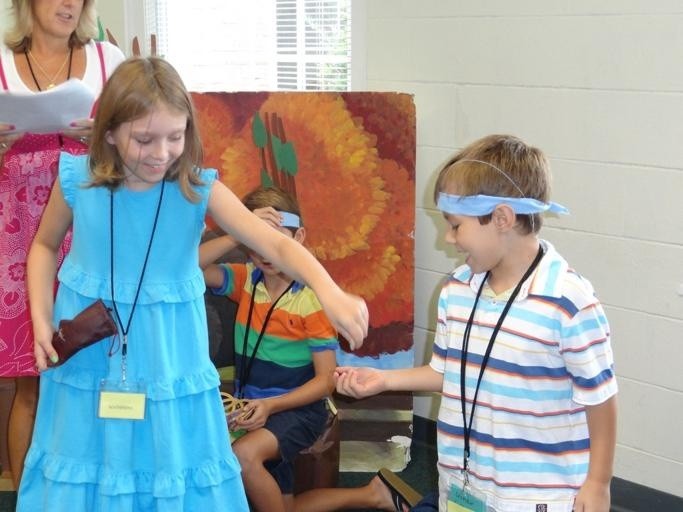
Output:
[376,467,424,512]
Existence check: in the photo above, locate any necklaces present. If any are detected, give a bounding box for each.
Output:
[27,43,74,89]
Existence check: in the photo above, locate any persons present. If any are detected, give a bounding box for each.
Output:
[15,54,370,510]
[327,132,625,511]
[0,0,131,495]
[196,186,432,512]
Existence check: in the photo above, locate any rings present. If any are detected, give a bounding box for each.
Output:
[78,136,88,143]
[2,142,9,151]
